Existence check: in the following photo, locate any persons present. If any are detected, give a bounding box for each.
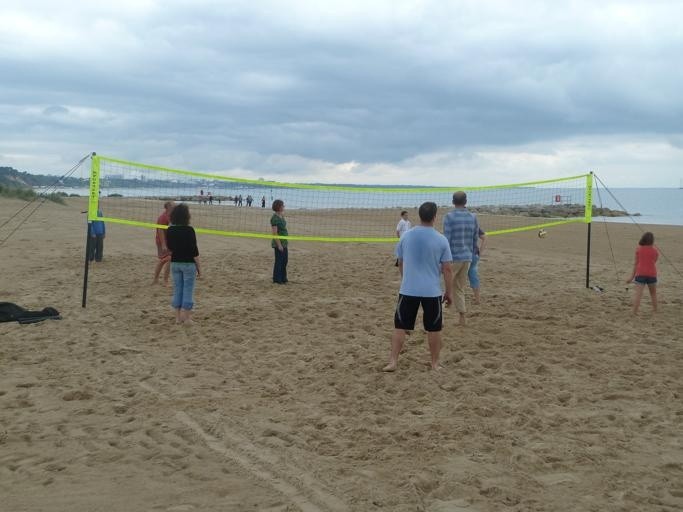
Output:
[381,201,453,374]
[467,226,486,304]
[625,233,658,316]
[87,202,105,262]
[270,199,289,284]
[443,191,480,327]
[198,188,265,210]
[150,200,175,287]
[163,204,201,325]
[394,210,410,266]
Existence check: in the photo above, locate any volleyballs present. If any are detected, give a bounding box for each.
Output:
[538,228,547,238]
